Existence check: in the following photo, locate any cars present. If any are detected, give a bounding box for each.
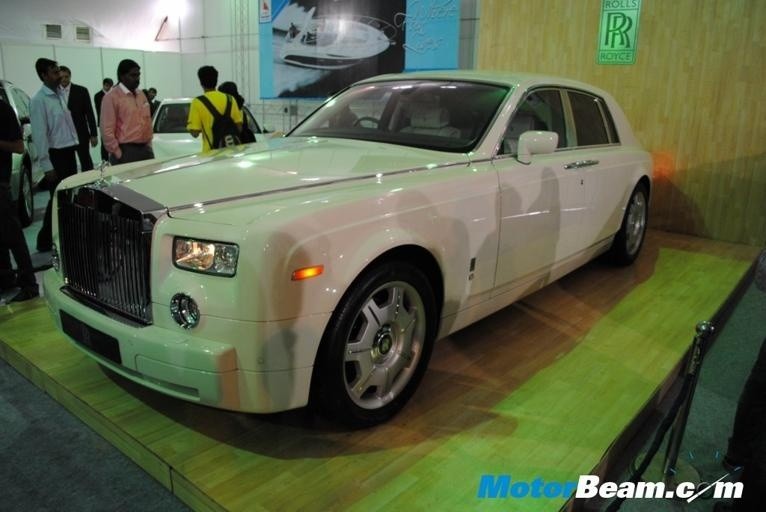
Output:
[0,78,48,228]
[37,70,653,434]
[151,97,276,160]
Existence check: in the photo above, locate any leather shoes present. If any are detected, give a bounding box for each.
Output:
[4,288,39,305]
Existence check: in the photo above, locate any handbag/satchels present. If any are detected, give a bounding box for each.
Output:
[242,112,257,144]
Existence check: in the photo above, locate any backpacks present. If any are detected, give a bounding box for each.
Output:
[195,94,241,149]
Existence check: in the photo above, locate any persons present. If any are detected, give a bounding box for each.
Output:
[29,58,81,253]
[59,65,98,175]
[142,88,157,117]
[99,59,155,166]
[0,98,38,302]
[216,82,261,134]
[186,66,246,152]
[94,78,113,166]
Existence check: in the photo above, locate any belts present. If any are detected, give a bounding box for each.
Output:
[120,142,146,147]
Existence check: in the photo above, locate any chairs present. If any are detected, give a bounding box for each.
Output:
[503,112,536,154]
[398,103,462,148]
